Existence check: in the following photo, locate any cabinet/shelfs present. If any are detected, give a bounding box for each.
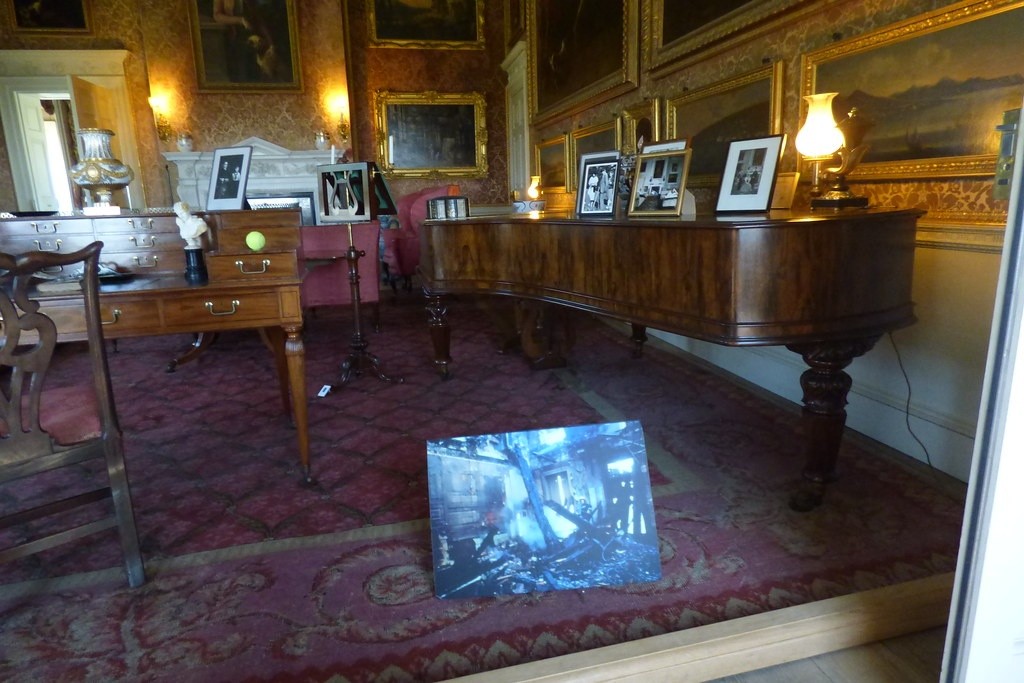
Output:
[0,204,314,483]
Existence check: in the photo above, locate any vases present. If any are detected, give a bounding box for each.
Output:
[68,127,133,205]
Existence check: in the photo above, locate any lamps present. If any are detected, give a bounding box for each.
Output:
[528,176,543,201]
[794,92,844,198]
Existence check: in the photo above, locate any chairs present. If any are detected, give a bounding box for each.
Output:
[0,240,145,594]
[383,183,448,291]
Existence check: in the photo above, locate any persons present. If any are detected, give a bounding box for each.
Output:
[219,161,229,198]
[231,166,240,198]
[196,0,293,82]
[588,168,615,211]
[172,202,208,249]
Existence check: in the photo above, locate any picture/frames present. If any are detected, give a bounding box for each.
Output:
[6,0,96,35]
[364,0,487,53]
[714,132,781,214]
[186,0,306,94]
[316,162,371,222]
[664,59,785,188]
[795,0,1024,183]
[206,144,253,210]
[534,96,692,218]
[370,87,488,181]
[524,0,639,128]
[639,0,813,75]
